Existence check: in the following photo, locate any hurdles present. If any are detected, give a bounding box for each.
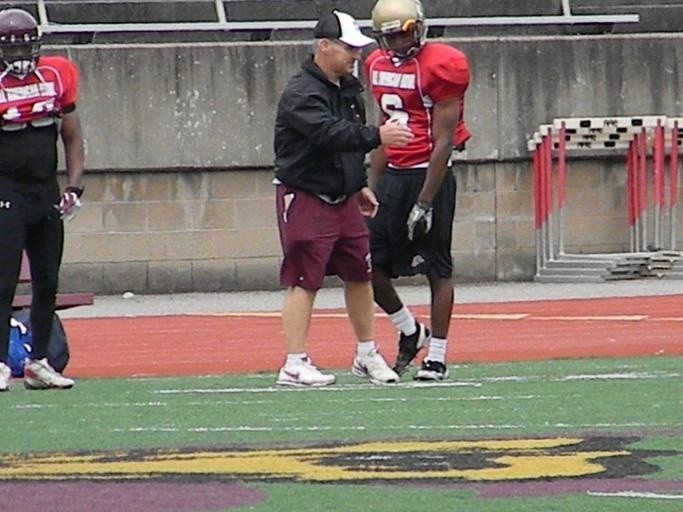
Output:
[527,116,683,283]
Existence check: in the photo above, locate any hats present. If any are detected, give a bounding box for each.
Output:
[315,11,376,46]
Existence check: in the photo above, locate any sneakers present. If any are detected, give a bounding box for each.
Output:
[277,357,336,390]
[0,361,12,391]
[392,322,429,377]
[351,345,399,385]
[416,355,449,381]
[21,355,76,389]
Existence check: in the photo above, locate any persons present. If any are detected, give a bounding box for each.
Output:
[272,9,416,385]
[364,1,471,383]
[0,9,86,392]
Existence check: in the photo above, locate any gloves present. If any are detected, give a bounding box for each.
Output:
[407,203,434,241]
[52,189,81,223]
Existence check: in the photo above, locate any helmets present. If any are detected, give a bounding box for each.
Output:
[372,0,427,59]
[0,8,39,76]
